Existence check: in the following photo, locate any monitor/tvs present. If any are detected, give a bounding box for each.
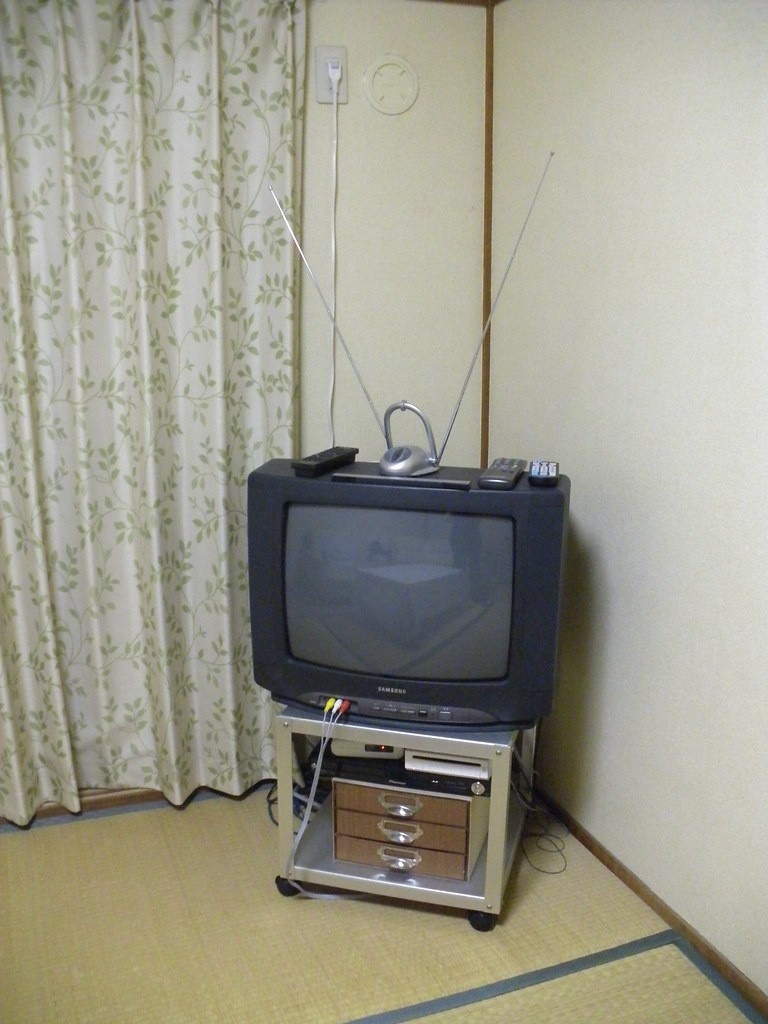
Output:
[247,458,572,732]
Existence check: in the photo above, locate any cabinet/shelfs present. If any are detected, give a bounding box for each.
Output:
[330,773,490,881]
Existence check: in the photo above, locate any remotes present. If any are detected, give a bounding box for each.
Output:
[527,461,559,488]
[290,446,359,478]
[478,458,528,490]
